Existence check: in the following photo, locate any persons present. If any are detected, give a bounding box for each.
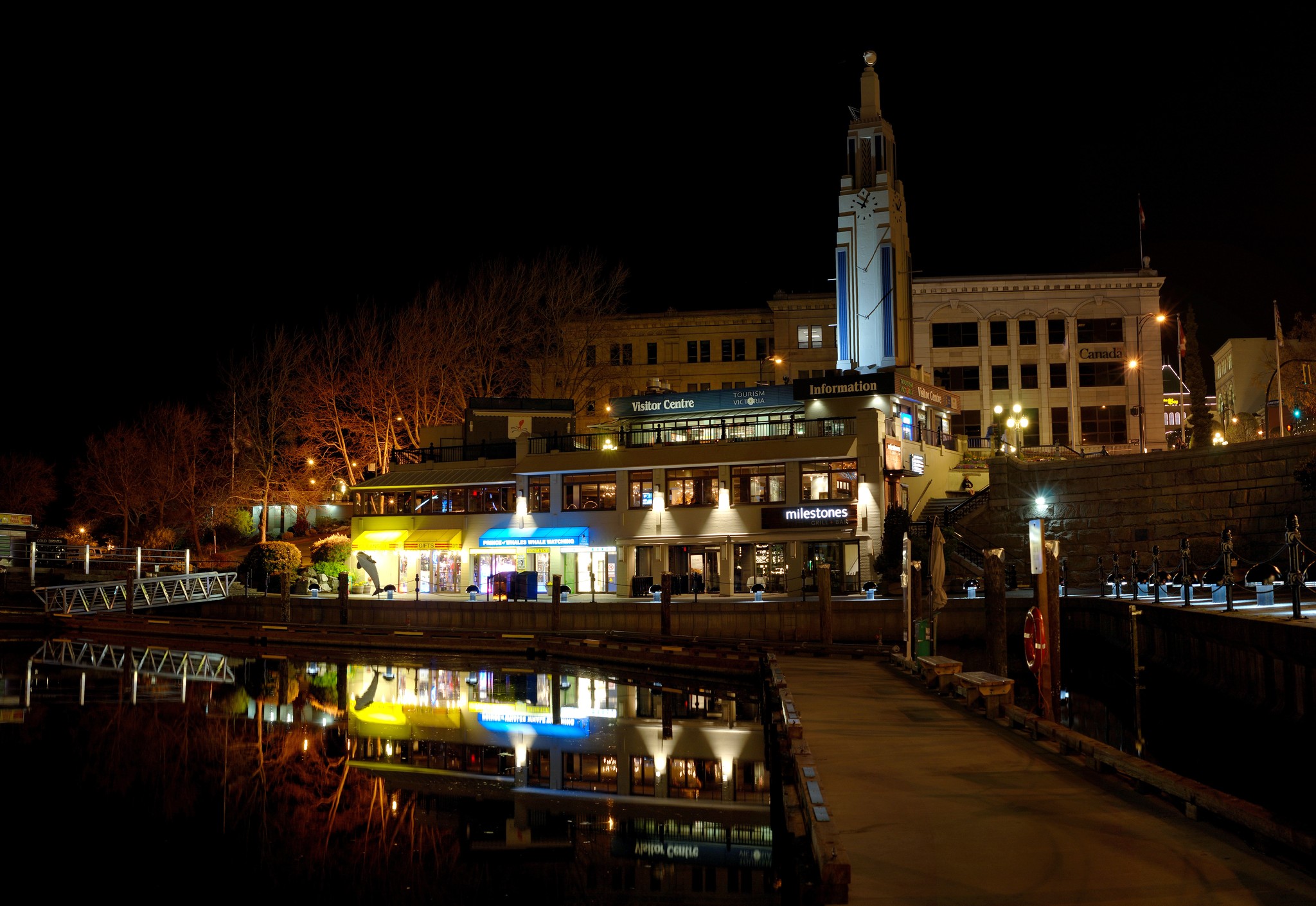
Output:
[1001,429,1014,454]
[984,425,995,447]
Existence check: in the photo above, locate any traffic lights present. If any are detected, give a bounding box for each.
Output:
[1287,364,1312,431]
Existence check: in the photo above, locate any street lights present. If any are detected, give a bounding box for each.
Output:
[1135,311,1169,453]
[994,404,1029,459]
[1213,403,1238,446]
[760,357,781,386]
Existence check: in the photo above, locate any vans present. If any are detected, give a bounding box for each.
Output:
[66,547,102,563]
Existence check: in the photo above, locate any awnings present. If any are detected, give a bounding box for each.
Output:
[478,713,590,739]
[351,530,408,550]
[478,527,590,546]
[402,705,461,731]
[404,529,462,550]
[349,700,405,726]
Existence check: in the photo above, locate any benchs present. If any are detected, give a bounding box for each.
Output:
[916,654,963,693]
[954,669,1015,718]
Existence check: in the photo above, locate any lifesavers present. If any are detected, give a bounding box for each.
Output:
[1024,605,1047,674]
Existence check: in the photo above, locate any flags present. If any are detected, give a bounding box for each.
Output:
[1179,320,1187,357]
[1277,307,1285,347]
[1139,200,1146,230]
